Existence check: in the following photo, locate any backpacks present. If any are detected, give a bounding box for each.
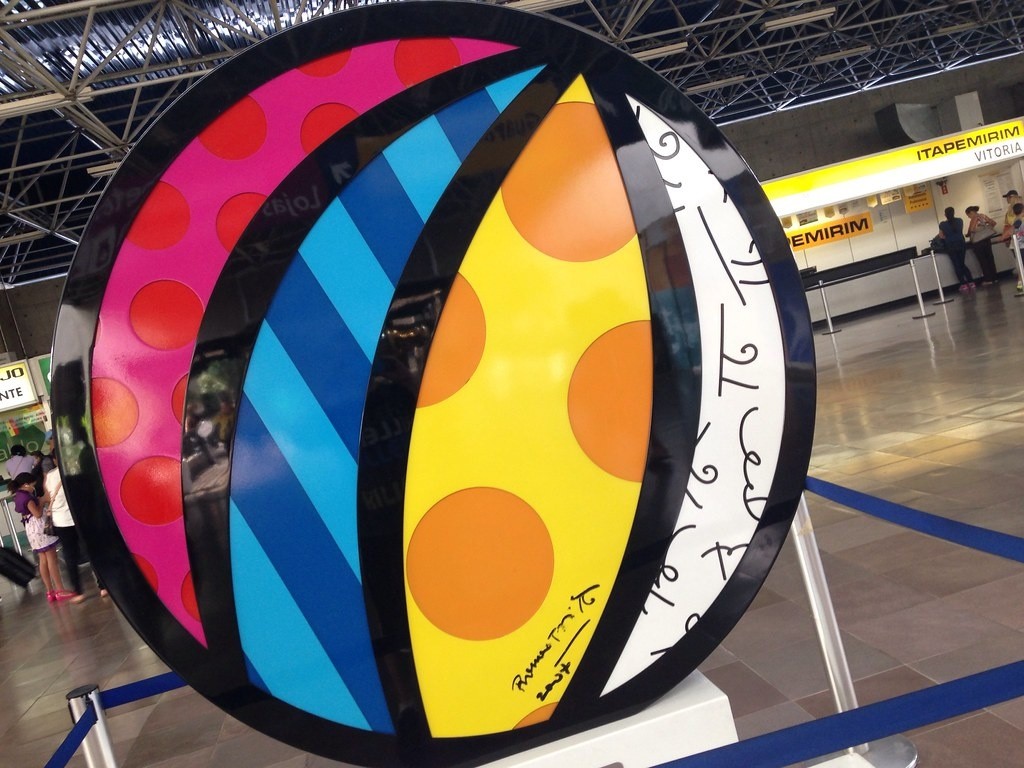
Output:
[929,233,946,254]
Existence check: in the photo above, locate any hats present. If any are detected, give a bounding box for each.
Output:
[1002,189,1018,198]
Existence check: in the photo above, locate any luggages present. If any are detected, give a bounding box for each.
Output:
[0,547,37,592]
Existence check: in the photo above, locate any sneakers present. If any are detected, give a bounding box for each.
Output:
[958,284,968,290]
[967,282,975,288]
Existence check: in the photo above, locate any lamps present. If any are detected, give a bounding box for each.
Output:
[935,22,978,34]
[685,75,745,93]
[87,162,121,178]
[815,45,871,62]
[0,86,96,119]
[760,7,836,32]
[0,231,46,247]
[632,42,689,61]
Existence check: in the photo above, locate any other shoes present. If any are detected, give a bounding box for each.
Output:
[993,280,1000,285]
[982,280,993,286]
[1016,280,1023,290]
[54,590,77,601]
[46,590,56,603]
[69,594,88,605]
[100,588,108,598]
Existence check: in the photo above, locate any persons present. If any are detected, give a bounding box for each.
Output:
[184,400,236,458]
[938,190,1024,296]
[3,434,110,602]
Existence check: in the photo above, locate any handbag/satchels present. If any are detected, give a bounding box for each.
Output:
[970,215,997,245]
[1002,224,1011,248]
[43,508,59,536]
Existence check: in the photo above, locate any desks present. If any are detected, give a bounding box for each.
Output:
[808,235,1016,322]
